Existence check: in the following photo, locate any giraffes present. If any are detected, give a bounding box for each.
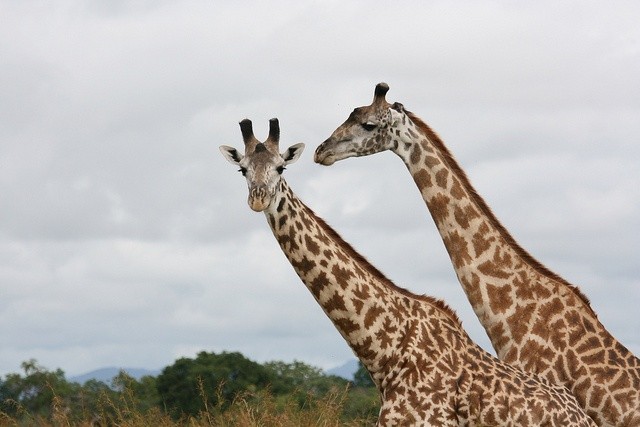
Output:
[313,81,640,427]
[218,118,599,427]
